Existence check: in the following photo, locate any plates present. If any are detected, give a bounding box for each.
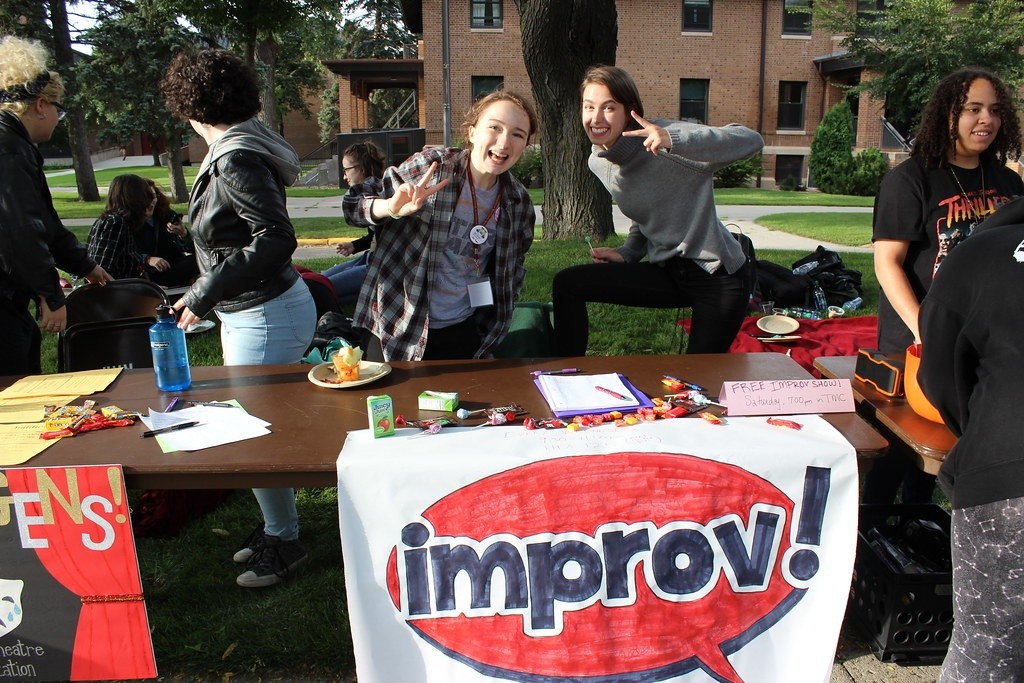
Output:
[188,320,215,334]
[757,316,799,334]
[308,362,392,388]
[758,336,802,342]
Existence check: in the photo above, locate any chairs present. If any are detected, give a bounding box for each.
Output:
[294,264,367,360]
[491,303,555,359]
[668,231,759,355]
[57,279,172,373]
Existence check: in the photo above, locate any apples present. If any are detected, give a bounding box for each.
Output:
[378,418,390,431]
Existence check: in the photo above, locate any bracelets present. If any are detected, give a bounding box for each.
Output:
[386,198,403,219]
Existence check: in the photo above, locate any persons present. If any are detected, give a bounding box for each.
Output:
[83,174,216,334]
[352,90,539,361]
[0,34,116,377]
[159,42,317,587]
[321,139,395,317]
[871,69,1024,683]
[551,65,766,357]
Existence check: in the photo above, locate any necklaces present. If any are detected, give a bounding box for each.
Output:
[949,161,985,223]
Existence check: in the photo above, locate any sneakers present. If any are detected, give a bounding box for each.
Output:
[232,529,266,563]
[236,533,306,587]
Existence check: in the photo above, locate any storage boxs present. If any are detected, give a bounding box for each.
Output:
[847,505,954,666]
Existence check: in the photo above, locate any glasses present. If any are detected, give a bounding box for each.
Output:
[342,165,360,175]
[46,100,68,121]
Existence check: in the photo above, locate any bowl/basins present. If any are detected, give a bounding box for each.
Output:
[760,301,774,314]
[904,343,945,424]
[772,308,788,317]
[827,306,844,319]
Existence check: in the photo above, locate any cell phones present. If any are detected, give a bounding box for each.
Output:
[171,213,184,226]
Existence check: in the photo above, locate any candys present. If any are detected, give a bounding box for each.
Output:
[585,236,591,242]
[39,399,144,439]
[393,377,802,435]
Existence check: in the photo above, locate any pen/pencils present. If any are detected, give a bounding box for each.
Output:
[530,368,582,376]
[595,385,627,400]
[139,421,200,439]
[662,375,702,391]
[187,400,233,408]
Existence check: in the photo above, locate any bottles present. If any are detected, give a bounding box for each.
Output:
[150,305,192,393]
[786,307,821,320]
[813,280,826,310]
[840,297,863,312]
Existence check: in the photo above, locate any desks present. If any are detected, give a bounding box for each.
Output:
[813,355,960,474]
[1,350,890,503]
